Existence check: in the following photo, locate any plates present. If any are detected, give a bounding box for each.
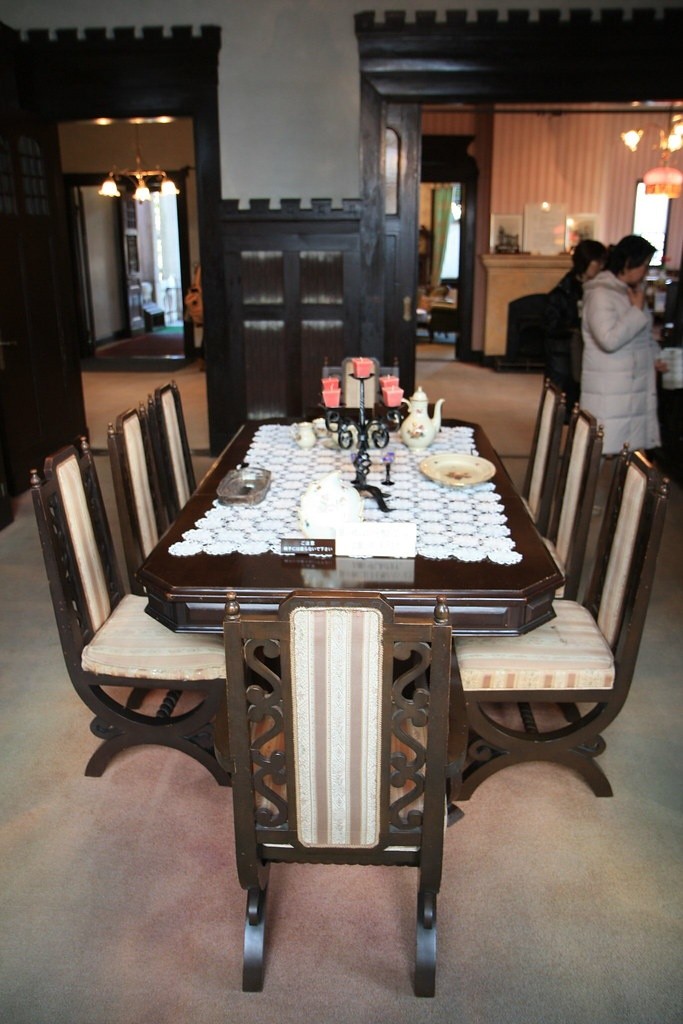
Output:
[418,454,497,489]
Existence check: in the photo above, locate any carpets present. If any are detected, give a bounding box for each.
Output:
[97,335,184,356]
[153,326,183,334]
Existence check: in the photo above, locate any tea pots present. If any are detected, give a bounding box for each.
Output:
[398,386,446,455]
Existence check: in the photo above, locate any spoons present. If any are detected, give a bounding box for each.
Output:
[381,463,394,486]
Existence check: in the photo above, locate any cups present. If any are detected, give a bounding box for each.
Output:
[287,418,379,452]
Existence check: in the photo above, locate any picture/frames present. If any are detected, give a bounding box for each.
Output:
[489,213,524,254]
[566,213,598,255]
[522,201,567,255]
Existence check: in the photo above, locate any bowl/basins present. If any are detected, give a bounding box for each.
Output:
[217,466,272,507]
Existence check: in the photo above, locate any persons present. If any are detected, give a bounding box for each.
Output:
[579,235,661,515]
[544,240,607,425]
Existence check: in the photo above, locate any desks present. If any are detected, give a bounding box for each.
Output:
[428,297,458,344]
[134,418,566,829]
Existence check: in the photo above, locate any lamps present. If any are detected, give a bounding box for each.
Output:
[98,123,181,203]
[622,101,683,197]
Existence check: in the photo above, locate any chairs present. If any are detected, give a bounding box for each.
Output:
[31,379,231,787]
[416,312,431,340]
[222,589,452,998]
[322,354,401,419]
[452,377,670,801]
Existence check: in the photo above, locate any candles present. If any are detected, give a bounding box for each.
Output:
[322,388,341,406]
[381,385,404,407]
[379,374,400,388]
[353,358,373,378]
[321,375,338,390]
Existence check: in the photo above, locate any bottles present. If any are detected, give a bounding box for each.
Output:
[651,256,671,314]
